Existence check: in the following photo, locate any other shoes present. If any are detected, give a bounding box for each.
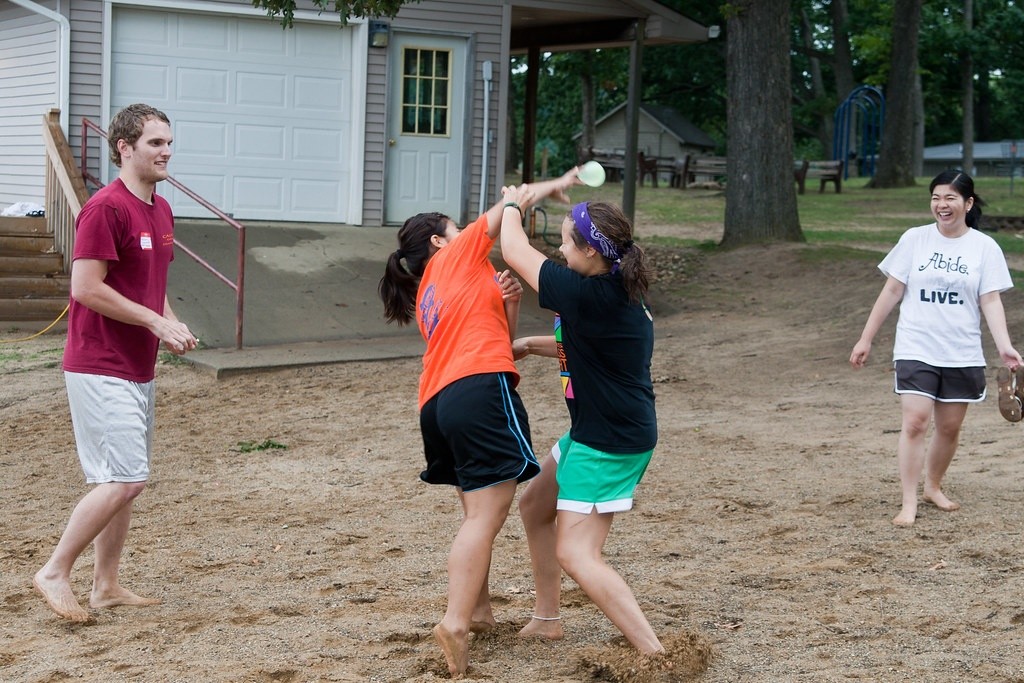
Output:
[996,366,1024,422]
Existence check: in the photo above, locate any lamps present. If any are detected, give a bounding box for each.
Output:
[368,20,390,49]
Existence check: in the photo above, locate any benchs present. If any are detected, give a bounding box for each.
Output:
[793,160,844,193]
[684,153,730,191]
[587,145,658,188]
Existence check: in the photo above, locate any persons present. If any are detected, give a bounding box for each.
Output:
[487,179,667,662]
[379,167,585,677]
[848,168,1024,526]
[30,102,200,623]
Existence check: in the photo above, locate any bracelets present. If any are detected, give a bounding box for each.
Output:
[503,201,524,216]
